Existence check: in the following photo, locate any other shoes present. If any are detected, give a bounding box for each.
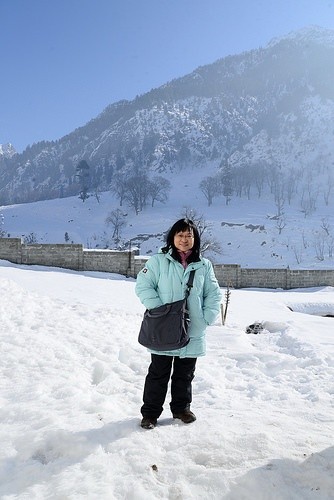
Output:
[172,409,196,423]
[140,417,157,428]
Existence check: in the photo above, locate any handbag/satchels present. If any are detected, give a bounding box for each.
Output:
[138,298,191,352]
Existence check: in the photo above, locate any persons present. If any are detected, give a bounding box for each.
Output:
[134,218,221,430]
